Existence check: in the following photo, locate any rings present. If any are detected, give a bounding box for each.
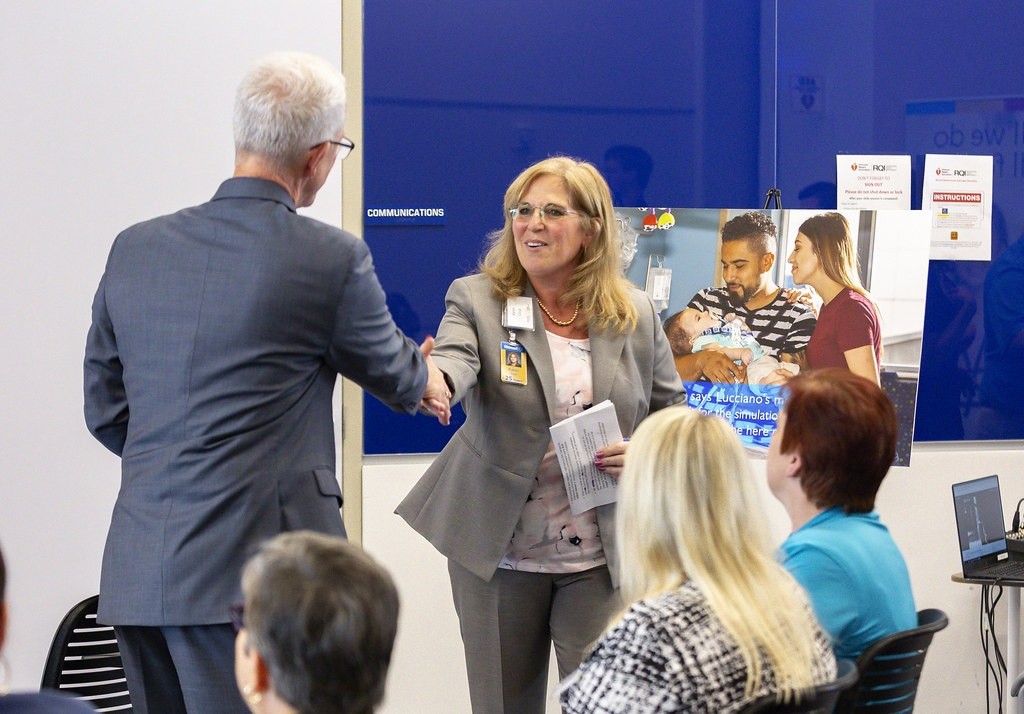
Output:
[445,391,448,396]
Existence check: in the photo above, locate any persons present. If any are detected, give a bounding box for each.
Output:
[392,155,688,714]
[565,405,838,714]
[507,354,521,368]
[0,548,97,714]
[83,54,452,714]
[229,529,400,713]
[766,364,918,714]
[659,211,817,449]
[760,213,882,452]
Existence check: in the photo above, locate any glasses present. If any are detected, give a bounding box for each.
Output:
[309,134,355,160]
[508,201,590,224]
[228,604,244,630]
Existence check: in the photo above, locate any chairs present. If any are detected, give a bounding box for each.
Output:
[737,609,948,714]
[40,595,136,714]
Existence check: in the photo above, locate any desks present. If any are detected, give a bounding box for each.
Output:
[951,572,1024,714]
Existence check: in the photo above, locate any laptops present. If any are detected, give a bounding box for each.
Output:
[951,474,1024,582]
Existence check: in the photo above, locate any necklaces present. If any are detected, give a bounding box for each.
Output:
[535,296,580,325]
